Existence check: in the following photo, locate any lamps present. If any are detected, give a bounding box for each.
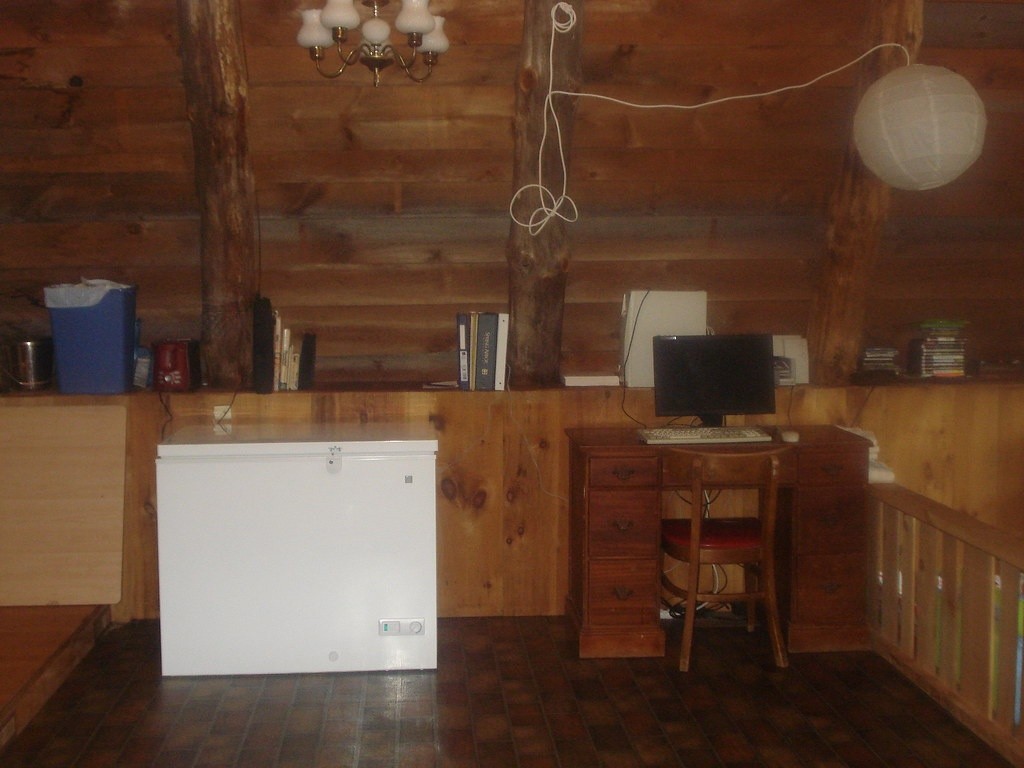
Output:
[296,0,450,84]
[850,63,989,191]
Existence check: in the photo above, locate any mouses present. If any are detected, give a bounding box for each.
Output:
[781,430,799,443]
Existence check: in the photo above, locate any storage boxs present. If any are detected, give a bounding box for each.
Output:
[45,282,139,394]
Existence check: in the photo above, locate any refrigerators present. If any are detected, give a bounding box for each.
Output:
[154,423,439,677]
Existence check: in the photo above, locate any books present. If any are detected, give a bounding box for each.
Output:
[772,335,810,384]
[561,372,620,387]
[468,312,509,391]
[273,311,300,392]
[862,318,972,377]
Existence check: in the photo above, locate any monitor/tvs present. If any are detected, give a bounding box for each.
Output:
[652,332,776,428]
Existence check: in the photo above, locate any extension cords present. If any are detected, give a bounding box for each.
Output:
[660,608,681,620]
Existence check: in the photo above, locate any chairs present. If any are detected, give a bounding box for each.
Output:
[664,449,791,670]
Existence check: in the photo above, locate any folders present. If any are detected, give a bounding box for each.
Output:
[456,311,509,390]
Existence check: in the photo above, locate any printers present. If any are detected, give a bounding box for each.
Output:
[772,334,809,386]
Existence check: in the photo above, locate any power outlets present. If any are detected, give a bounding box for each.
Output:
[213,404,232,420]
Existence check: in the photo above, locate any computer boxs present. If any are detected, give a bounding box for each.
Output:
[618,290,707,387]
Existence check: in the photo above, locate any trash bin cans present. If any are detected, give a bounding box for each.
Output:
[44,282,139,393]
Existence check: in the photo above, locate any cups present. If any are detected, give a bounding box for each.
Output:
[10,337,52,393]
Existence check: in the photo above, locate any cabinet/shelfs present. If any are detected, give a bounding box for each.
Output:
[566,424,879,659]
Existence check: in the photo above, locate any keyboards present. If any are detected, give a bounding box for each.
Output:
[636,427,771,445]
